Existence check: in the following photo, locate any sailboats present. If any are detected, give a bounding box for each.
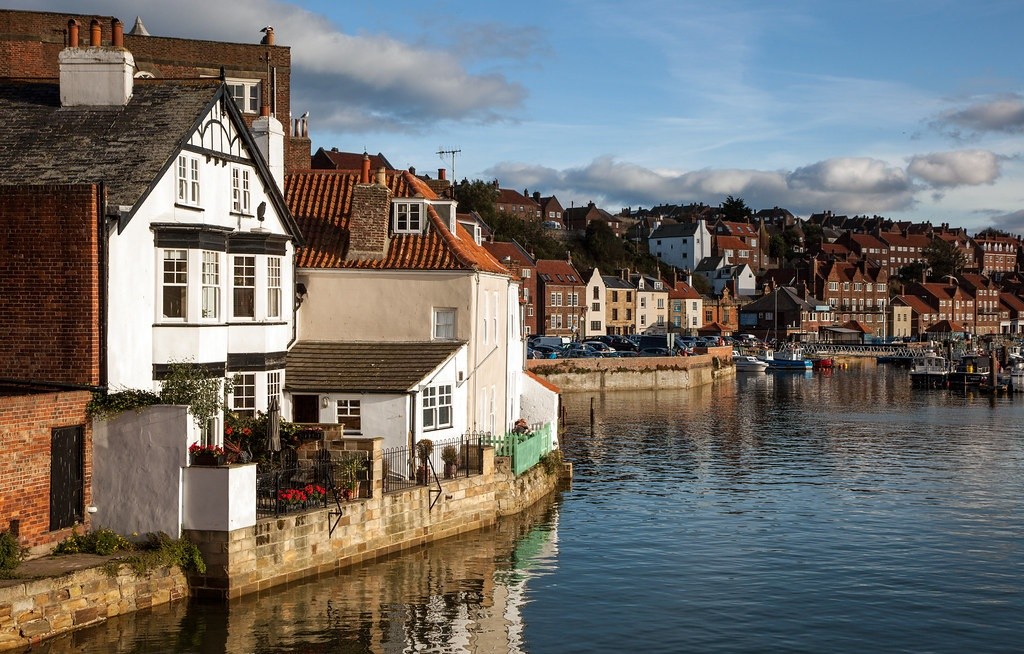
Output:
[755,288,814,370]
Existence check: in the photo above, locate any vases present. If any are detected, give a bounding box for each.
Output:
[191,454,228,467]
[237,447,254,464]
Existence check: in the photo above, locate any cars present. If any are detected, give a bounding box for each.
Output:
[524,328,785,359]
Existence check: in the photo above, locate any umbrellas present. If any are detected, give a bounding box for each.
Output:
[268,399,282,497]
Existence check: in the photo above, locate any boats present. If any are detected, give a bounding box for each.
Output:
[905,336,1024,396]
[809,357,832,368]
[731,350,769,372]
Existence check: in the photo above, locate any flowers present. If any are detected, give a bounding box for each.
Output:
[188,439,226,459]
[274,481,327,511]
[223,412,254,451]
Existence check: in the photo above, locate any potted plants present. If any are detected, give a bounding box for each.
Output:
[439,444,460,480]
[330,452,369,500]
[406,439,435,486]
